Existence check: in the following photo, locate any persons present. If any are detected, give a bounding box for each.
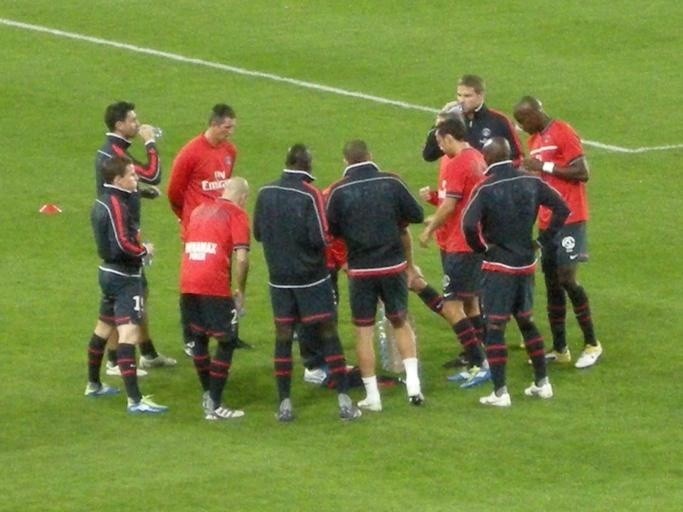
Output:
[420,113,484,369]
[253,143,364,424]
[414,120,490,390]
[329,139,425,409]
[511,96,603,371]
[462,137,569,409]
[93,103,177,380]
[180,176,251,422]
[295,187,353,385]
[423,72,526,168]
[167,103,253,361]
[399,227,444,320]
[84,159,169,415]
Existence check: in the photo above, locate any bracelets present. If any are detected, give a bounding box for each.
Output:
[541,161,556,176]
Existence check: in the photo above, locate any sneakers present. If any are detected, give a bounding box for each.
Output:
[105,359,148,376]
[83,381,123,397]
[522,381,554,398]
[302,367,328,384]
[124,394,170,414]
[338,404,362,420]
[478,390,512,407]
[445,365,480,381]
[442,351,470,369]
[527,348,572,364]
[230,338,254,351]
[203,405,245,421]
[458,367,492,388]
[573,340,603,368]
[405,389,426,406]
[181,341,195,357]
[276,398,294,422]
[356,396,384,411]
[200,389,214,414]
[138,352,178,369]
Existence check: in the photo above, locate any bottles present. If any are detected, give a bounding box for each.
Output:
[137,124,162,137]
[447,102,464,115]
[141,240,153,268]
